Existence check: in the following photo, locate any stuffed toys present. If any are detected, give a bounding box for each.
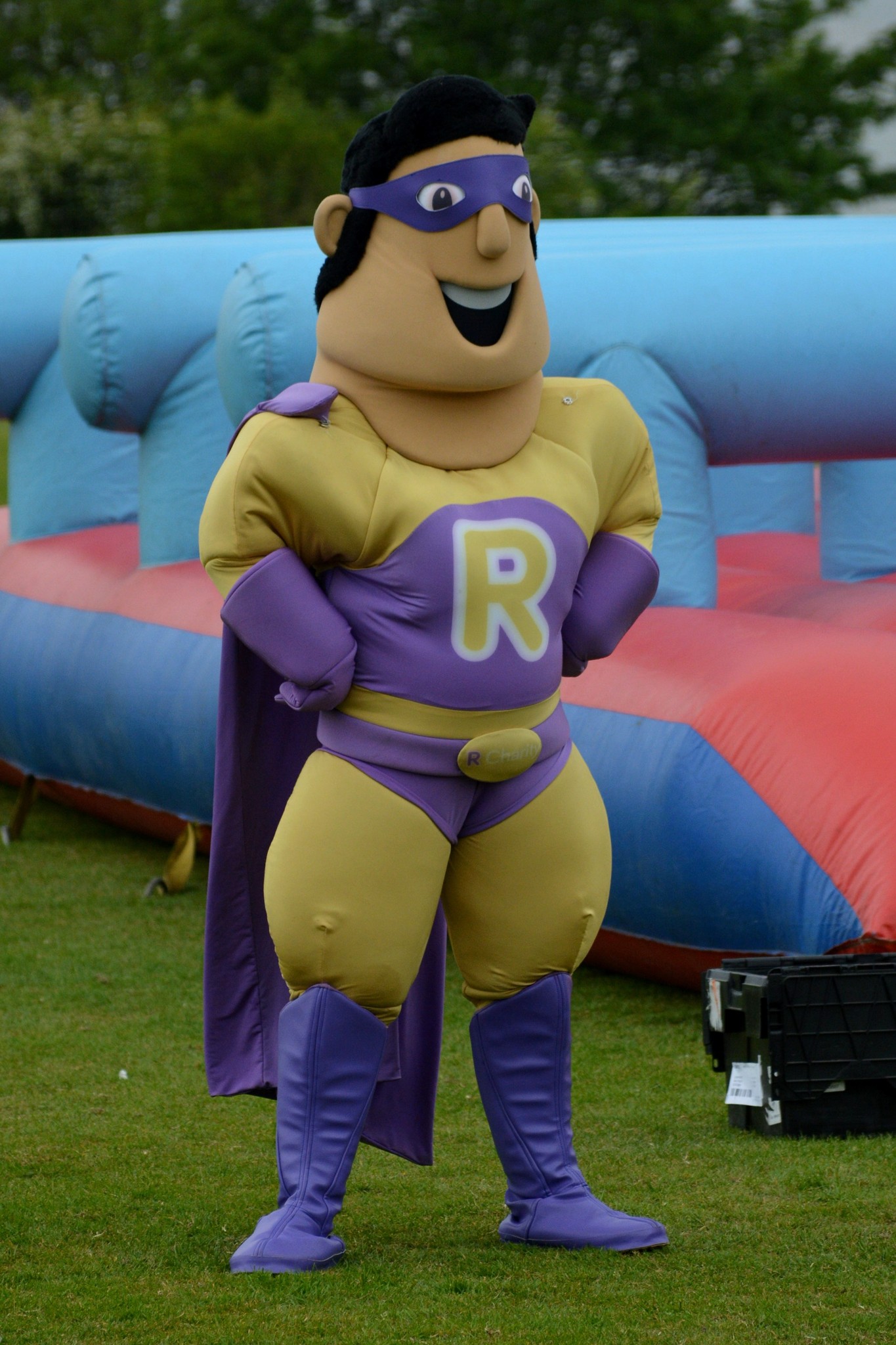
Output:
[197,81,671,1274]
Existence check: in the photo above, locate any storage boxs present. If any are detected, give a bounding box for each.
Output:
[701,953,896,1139]
